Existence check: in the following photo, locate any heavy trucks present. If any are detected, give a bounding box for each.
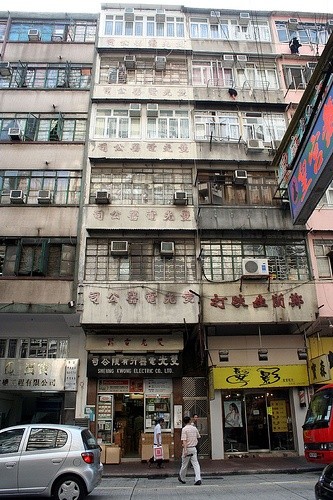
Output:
[302,384,333,465]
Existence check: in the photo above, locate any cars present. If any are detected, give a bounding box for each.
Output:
[314,464,333,500]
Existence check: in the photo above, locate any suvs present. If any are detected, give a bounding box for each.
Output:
[0,424,102,500]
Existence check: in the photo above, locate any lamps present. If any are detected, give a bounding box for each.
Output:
[219,349,308,361]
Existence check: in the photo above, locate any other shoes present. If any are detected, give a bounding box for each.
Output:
[178,477,186,484]
[194,480,201,485]
[157,466,164,469]
[147,459,151,469]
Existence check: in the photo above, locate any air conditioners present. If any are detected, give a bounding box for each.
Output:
[305,62,318,70]
[110,241,176,254]
[222,55,247,67]
[95,192,109,203]
[0,61,13,76]
[210,11,250,24]
[128,103,159,117]
[124,8,165,22]
[175,192,187,204]
[8,128,22,139]
[241,259,269,277]
[288,18,333,29]
[28,30,63,42]
[123,55,167,71]
[9,190,54,203]
[234,170,247,185]
[246,140,281,152]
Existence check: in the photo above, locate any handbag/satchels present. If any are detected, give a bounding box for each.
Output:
[153,445,164,461]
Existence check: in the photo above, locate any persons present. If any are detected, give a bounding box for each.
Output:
[148,418,165,469]
[178,416,201,486]
[191,414,202,457]
[224,403,242,427]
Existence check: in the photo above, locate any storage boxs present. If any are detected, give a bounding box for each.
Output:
[99,443,121,464]
[141,433,171,460]
